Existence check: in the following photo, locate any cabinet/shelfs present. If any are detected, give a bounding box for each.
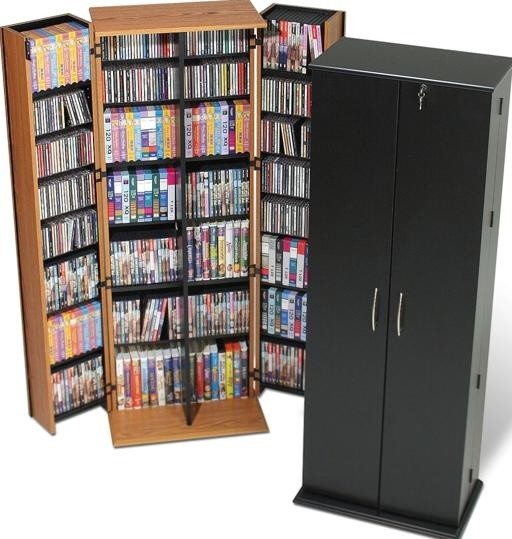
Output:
[303,37,512,524]
[1,4,347,447]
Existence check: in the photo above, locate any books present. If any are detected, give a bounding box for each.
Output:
[188,338,249,404]
[47,299,104,369]
[110,229,183,287]
[50,352,106,416]
[32,88,92,136]
[261,112,312,161]
[186,217,251,284]
[185,164,251,219]
[103,104,177,163]
[112,293,183,345]
[184,100,251,158]
[183,29,251,56]
[101,59,178,104]
[187,285,250,339]
[107,166,182,225]
[184,56,251,98]
[19,20,91,94]
[114,342,187,411]
[260,338,306,392]
[41,206,99,260]
[261,286,308,342]
[260,233,309,289]
[44,249,102,313]
[261,153,311,200]
[38,167,96,220]
[260,194,309,239]
[35,126,95,178]
[261,71,312,119]
[100,34,178,61]
[262,8,331,75]
